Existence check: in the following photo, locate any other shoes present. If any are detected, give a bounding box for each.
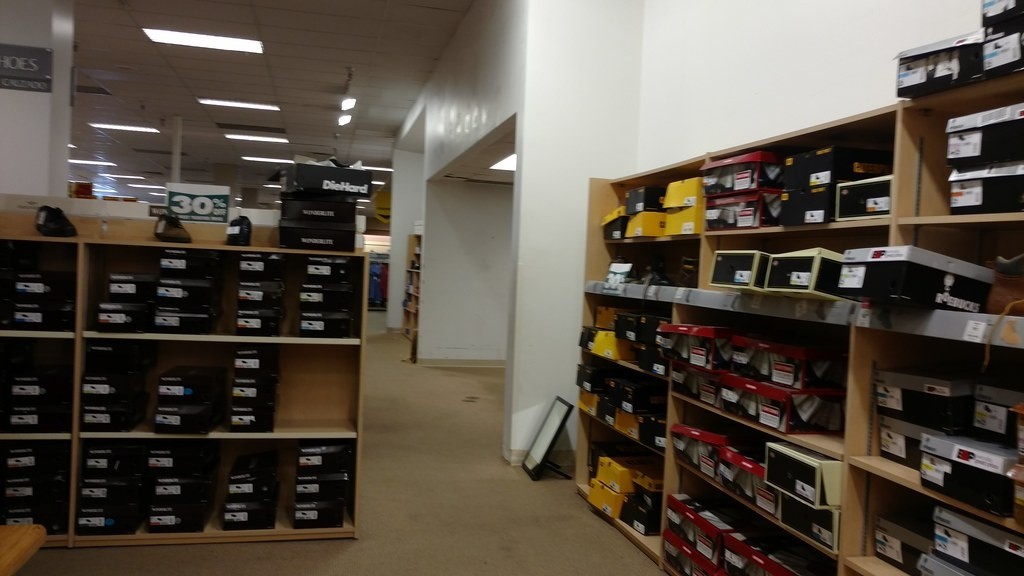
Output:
[34,205,79,237]
[153,213,192,242]
[410,260,420,269]
[225,216,252,246]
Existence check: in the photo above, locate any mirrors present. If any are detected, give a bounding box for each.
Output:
[521,396,574,481]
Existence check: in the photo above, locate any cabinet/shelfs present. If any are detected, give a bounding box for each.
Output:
[574,74,1024,576]
[0,211,371,549]
[402,233,422,341]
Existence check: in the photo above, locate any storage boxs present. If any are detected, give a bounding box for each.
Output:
[606,262,638,282]
[663,494,838,576]
[700,143,892,230]
[600,177,704,240]
[580,306,671,376]
[1,248,360,536]
[874,366,1024,523]
[709,245,997,313]
[656,324,850,435]
[278,164,373,252]
[670,423,845,555]
[946,101,1024,214]
[586,445,665,535]
[895,0,1024,99]
[576,363,668,449]
[872,503,1024,576]
